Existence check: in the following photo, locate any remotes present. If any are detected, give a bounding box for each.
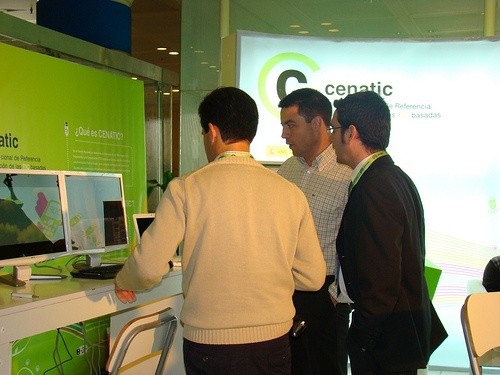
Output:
[0,274,26,286]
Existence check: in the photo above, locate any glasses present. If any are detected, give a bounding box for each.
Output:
[326,126,342,134]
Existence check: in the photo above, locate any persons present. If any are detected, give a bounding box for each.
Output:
[115,87,327,375]
[275,87,353,375]
[326,92,449,374]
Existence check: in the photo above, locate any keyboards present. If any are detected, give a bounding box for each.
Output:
[70,264,124,280]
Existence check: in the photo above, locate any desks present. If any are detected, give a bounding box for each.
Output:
[0,255,185,375]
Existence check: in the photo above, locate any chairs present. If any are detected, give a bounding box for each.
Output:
[460,291,500,375]
[105,307,177,375]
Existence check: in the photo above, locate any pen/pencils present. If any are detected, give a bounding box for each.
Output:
[11,292,39,299]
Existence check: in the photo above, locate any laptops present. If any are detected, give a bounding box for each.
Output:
[133,213,182,267]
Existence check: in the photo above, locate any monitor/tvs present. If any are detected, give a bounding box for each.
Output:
[61,171,129,270]
[0,168,70,281]
